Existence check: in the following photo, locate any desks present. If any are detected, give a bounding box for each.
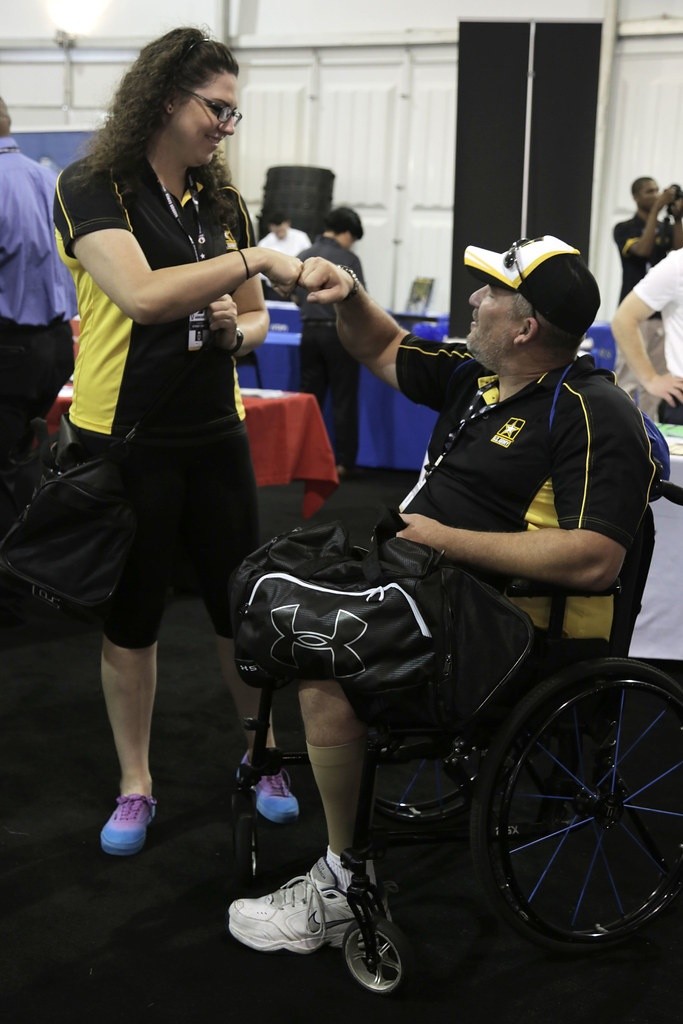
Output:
[46,297,682,662]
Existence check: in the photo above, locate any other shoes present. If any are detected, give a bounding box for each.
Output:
[338,466,350,480]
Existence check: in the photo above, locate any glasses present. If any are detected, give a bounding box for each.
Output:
[503,238,536,322]
[172,83,242,127]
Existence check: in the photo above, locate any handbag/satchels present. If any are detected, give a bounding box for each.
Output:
[232,511,535,747]
[0,416,138,623]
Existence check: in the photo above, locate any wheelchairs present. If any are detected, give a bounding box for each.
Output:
[233,433,682,999]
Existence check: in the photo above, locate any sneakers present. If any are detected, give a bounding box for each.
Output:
[227,857,392,954]
[101,794,157,855]
[237,755,300,825]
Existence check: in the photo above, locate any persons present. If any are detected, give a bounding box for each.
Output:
[227,233,654,953]
[255,210,312,302]
[609,246,683,427]
[196,330,203,341]
[291,205,366,480]
[616,177,683,421]
[53,27,303,855]
[0,99,80,634]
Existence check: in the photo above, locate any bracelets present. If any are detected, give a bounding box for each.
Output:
[214,331,243,354]
[239,250,250,279]
[336,264,359,302]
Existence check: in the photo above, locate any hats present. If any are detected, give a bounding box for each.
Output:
[464,236,601,337]
